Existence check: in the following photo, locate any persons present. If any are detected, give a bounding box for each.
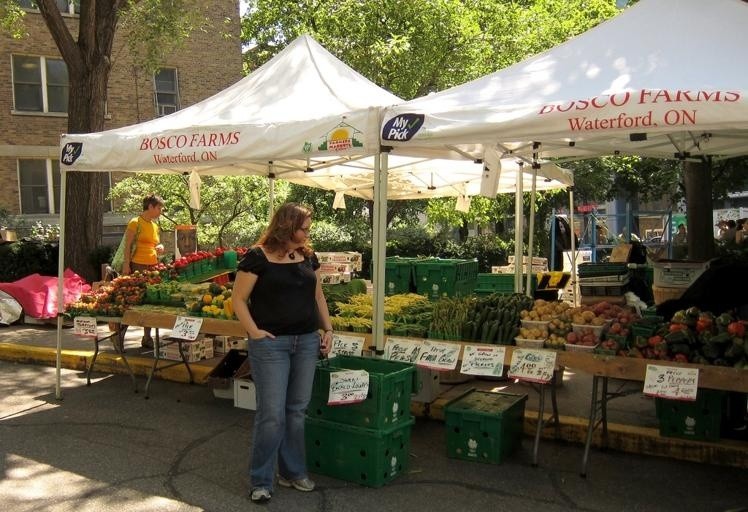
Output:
[111,194,166,355]
[618,227,640,242]
[716,218,747,250]
[674,224,688,246]
[231,203,334,503]
[177,229,197,257]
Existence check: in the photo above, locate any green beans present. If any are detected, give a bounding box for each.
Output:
[392,296,469,341]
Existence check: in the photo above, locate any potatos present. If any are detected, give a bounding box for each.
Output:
[516,298,639,346]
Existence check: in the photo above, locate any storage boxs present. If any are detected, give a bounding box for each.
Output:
[314,251,363,284]
[654,257,718,289]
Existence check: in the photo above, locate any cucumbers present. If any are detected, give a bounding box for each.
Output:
[463,291,534,345]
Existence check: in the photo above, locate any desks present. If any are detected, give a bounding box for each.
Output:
[62,310,748,478]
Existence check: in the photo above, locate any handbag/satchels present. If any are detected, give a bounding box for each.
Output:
[111,224,142,272]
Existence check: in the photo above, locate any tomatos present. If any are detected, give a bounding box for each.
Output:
[153,246,248,270]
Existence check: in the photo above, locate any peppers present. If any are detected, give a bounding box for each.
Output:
[186,283,234,320]
[619,306,748,368]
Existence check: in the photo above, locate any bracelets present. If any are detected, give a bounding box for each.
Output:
[324,328,334,332]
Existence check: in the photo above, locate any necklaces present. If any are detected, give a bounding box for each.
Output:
[288,248,297,259]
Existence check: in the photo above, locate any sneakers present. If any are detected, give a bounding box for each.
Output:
[279,479,315,492]
[142,336,153,348]
[251,488,271,501]
[111,335,124,353]
